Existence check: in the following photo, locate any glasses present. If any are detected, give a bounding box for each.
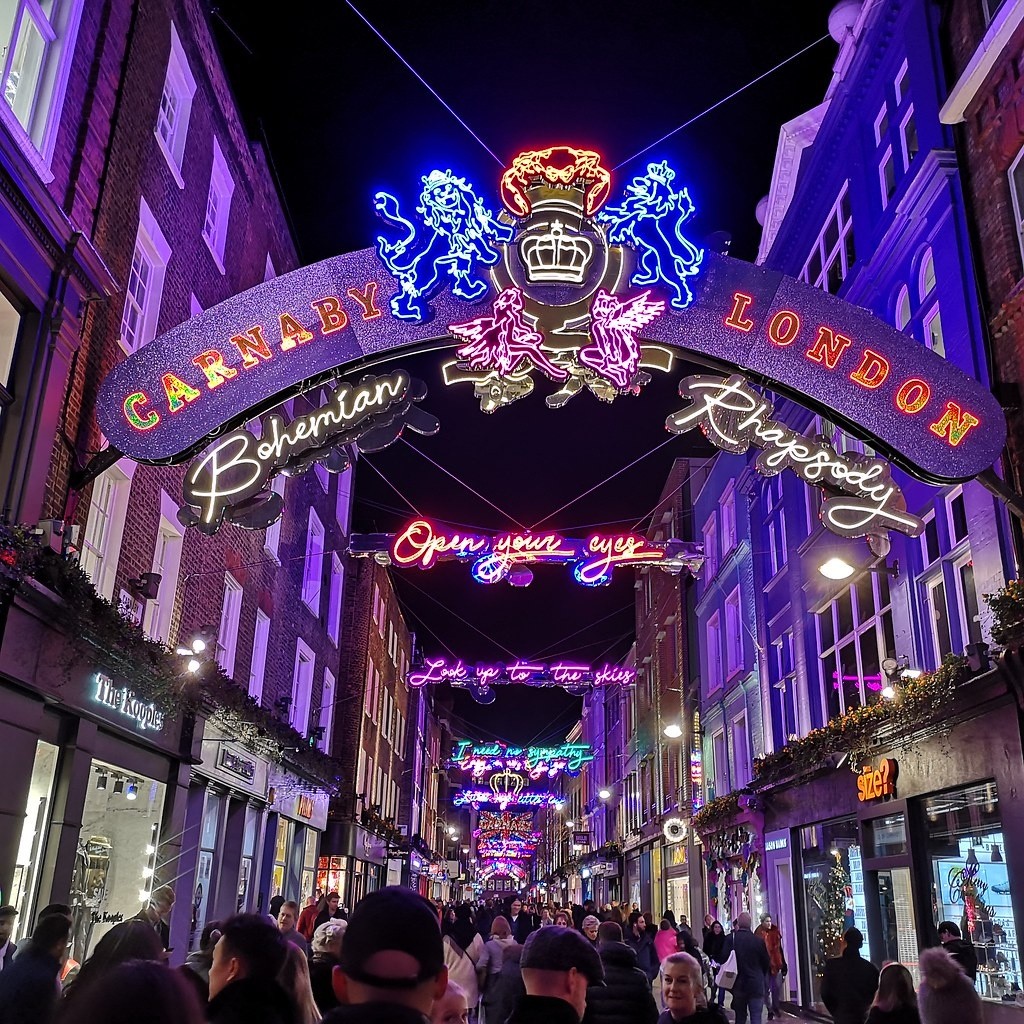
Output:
[66,941,72,947]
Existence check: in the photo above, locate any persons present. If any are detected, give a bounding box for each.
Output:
[654,919,678,963]
[0,885,994,1024]
[581,915,600,947]
[126,884,175,968]
[540,908,554,927]
[475,916,518,1005]
[314,892,347,931]
[64,837,111,964]
[276,901,307,954]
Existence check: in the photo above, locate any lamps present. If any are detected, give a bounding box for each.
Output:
[990,834,1003,862]
[881,658,910,681]
[663,725,706,738]
[96,773,108,790]
[310,726,326,740]
[281,781,341,798]
[28,519,62,556]
[274,697,293,714]
[818,557,898,580]
[632,818,659,836]
[566,820,582,827]
[129,572,163,599]
[964,642,990,675]
[113,778,124,795]
[357,793,395,823]
[127,783,138,800]
[966,837,978,864]
[175,634,215,657]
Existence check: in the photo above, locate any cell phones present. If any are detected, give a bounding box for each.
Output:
[165,948,175,959]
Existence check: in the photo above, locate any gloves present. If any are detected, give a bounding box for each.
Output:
[780,965,787,976]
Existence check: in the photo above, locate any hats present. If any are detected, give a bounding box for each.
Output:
[490,916,511,935]
[199,920,224,949]
[519,925,608,989]
[0,906,18,916]
[455,904,472,918]
[844,926,863,944]
[311,918,348,953]
[339,886,444,991]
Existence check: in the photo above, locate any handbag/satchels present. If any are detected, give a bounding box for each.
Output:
[715,931,739,989]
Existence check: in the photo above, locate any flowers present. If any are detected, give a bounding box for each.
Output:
[689,787,753,834]
[751,652,975,802]
[980,578,1024,643]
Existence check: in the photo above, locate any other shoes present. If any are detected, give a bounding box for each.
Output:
[966,925,1024,1008]
[772,1008,780,1018]
[768,1012,774,1020]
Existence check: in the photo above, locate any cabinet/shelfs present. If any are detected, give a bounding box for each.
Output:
[968,918,1008,1000]
